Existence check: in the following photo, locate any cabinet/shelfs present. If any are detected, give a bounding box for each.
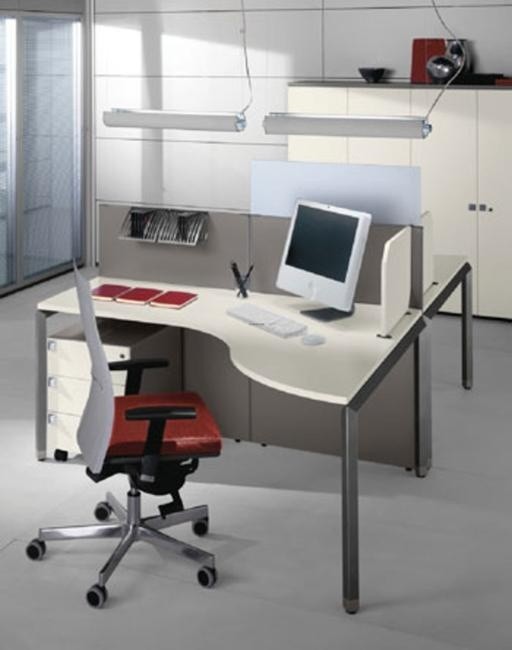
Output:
[285,81,511,320]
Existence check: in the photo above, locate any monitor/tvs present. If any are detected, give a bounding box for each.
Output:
[276,199,372,322]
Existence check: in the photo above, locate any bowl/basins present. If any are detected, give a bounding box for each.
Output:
[359,68,385,83]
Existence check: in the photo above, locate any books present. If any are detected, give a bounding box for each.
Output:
[151,290,198,308]
[116,286,164,305]
[92,283,130,300]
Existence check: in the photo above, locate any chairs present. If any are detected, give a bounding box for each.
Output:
[24,255,219,609]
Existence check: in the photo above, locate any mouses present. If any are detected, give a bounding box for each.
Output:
[301,334,327,346]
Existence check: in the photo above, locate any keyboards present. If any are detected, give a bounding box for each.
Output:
[227,303,307,339]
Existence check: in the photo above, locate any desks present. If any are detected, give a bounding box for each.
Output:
[33,161,473,615]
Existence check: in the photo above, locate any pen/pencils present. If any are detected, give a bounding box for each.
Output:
[231,262,253,297]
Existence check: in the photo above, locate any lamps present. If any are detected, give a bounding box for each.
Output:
[102,2,257,136]
[264,1,464,138]
